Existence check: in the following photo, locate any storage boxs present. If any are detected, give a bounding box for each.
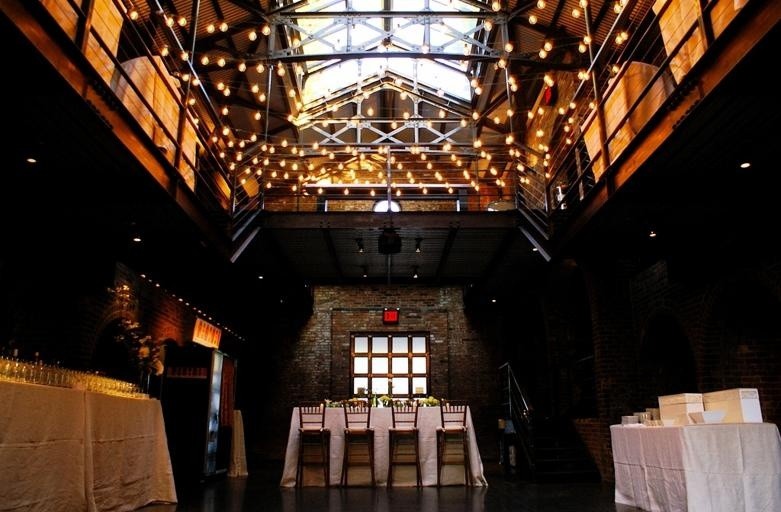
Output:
[657,387,762,423]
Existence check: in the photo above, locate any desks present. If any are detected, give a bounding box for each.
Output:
[1,379,178,511]
[610,423,781,512]
[280,405,488,487]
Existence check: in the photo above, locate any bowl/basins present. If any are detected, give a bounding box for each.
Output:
[688,410,725,425]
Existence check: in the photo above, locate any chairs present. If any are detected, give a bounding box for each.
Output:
[296,401,332,487]
[435,400,474,488]
[340,400,375,489]
[387,399,424,489]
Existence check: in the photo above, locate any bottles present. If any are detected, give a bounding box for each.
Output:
[0,355,150,400]
